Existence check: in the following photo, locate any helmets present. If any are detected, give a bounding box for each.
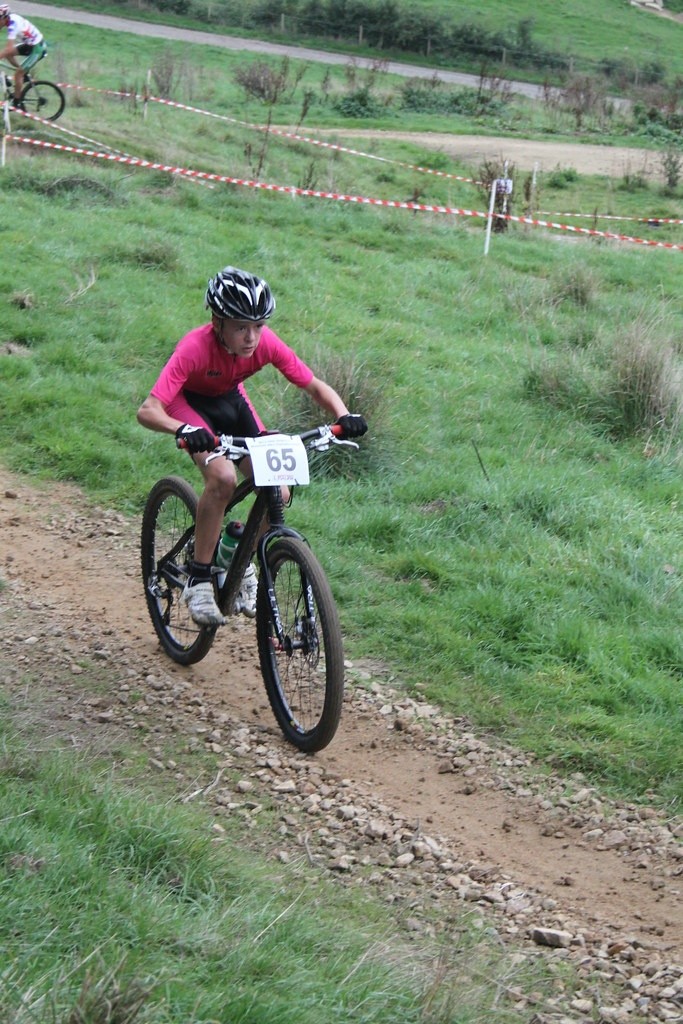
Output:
[0,5,10,19]
[204,266,275,321]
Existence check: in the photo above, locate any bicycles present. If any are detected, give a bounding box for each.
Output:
[0,60,66,121]
[140,424,360,754]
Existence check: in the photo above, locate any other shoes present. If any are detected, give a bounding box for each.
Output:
[0,100,23,111]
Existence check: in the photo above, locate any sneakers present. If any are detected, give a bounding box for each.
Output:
[226,562,258,617]
[181,578,222,626]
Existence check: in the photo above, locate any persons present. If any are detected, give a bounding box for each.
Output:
[137,265,367,622]
[0,4,47,112]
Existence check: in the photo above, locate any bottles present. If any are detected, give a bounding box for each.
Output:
[216,522,244,571]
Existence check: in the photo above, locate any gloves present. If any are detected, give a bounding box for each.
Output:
[177,425,212,452]
[336,414,368,439]
